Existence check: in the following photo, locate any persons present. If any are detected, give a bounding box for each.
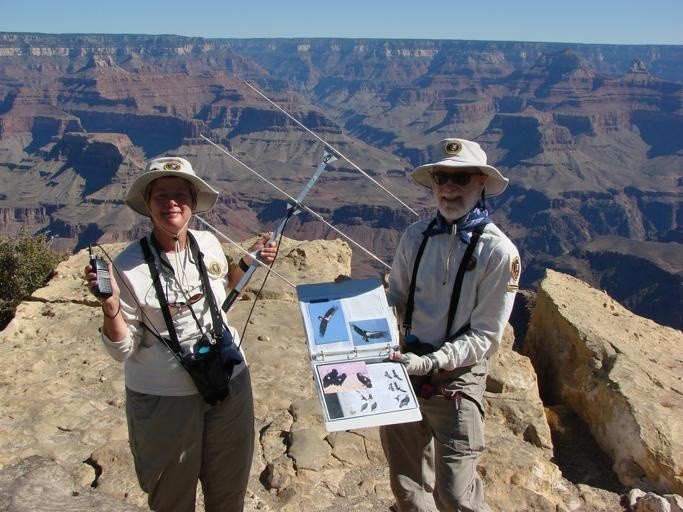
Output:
[84,156,277,511]
[384,138,523,512]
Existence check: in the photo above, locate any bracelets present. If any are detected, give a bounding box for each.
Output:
[425,352,439,377]
[238,257,250,273]
[101,300,120,319]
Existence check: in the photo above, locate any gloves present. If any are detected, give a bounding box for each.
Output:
[390,349,433,376]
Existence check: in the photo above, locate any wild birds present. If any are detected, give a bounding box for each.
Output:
[352,324,388,342]
[317,305,339,338]
[323,368,411,416]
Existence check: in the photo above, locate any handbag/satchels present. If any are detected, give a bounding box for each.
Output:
[401,343,449,400]
[180,328,244,406]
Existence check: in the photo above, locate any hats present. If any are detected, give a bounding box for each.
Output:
[410,138,509,199]
[123,157,219,217]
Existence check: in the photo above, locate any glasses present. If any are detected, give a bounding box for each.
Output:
[166,271,205,308]
[430,172,483,185]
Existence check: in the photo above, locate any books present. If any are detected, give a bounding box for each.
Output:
[297,276,423,433]
